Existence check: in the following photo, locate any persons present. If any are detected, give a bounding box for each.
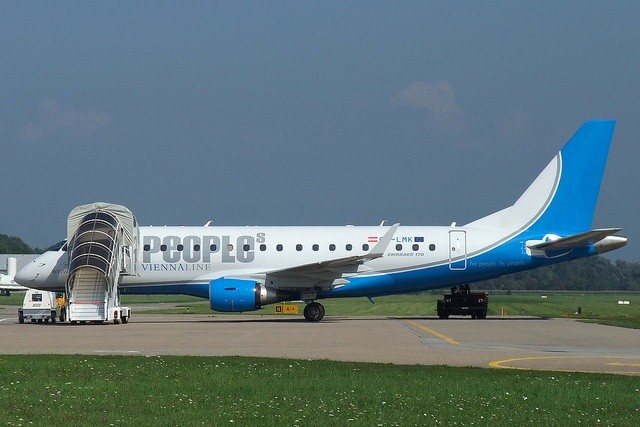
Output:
[55,294,67,320]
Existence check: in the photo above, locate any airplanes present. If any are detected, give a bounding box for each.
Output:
[0,118,629,322]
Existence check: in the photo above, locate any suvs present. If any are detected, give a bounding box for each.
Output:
[436,291,488,319]
[18,289,56,325]
